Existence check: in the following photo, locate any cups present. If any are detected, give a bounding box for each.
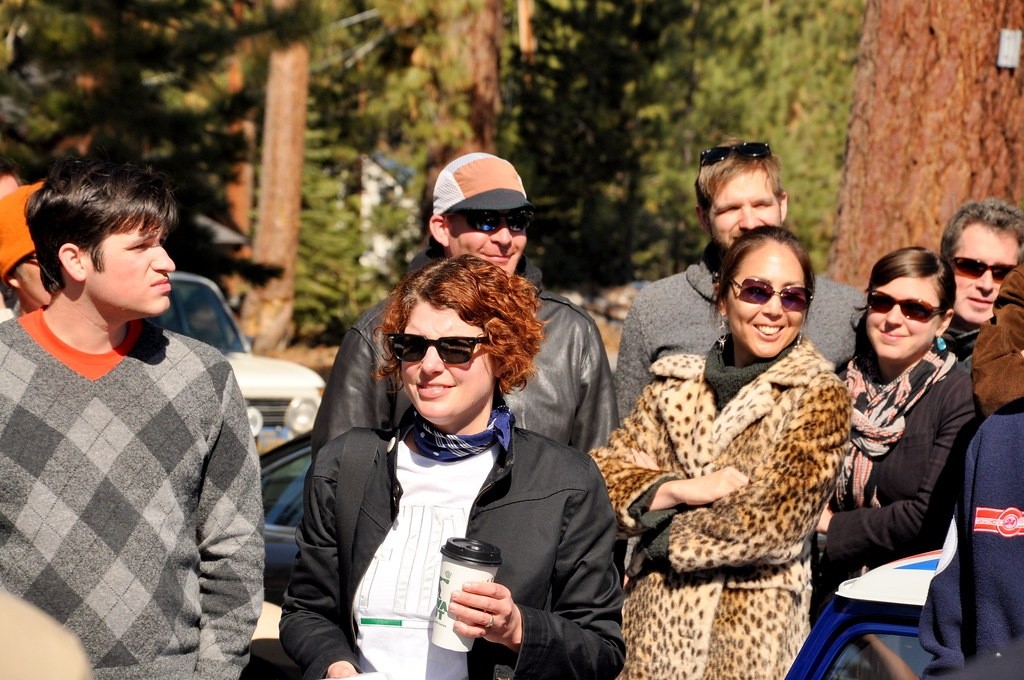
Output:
[430,537,503,653]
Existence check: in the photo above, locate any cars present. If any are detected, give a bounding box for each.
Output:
[136,270,325,471]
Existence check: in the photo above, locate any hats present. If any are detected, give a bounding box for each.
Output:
[0,181,46,289]
[432,151,535,216]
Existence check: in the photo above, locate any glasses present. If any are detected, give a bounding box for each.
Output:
[728,279,813,310]
[867,286,946,323]
[450,208,535,232]
[388,332,491,363]
[950,256,1019,283]
[700,143,774,166]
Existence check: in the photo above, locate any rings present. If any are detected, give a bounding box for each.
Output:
[485,616,494,628]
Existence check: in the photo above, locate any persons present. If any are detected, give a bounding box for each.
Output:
[809,245,977,629]
[941,197,1024,363]
[613,138,869,425]
[312,152,620,457]
[0,156,265,680]
[588,226,853,680]
[279,255,626,680]
[971,262,1024,419]
[919,398,1024,680]
